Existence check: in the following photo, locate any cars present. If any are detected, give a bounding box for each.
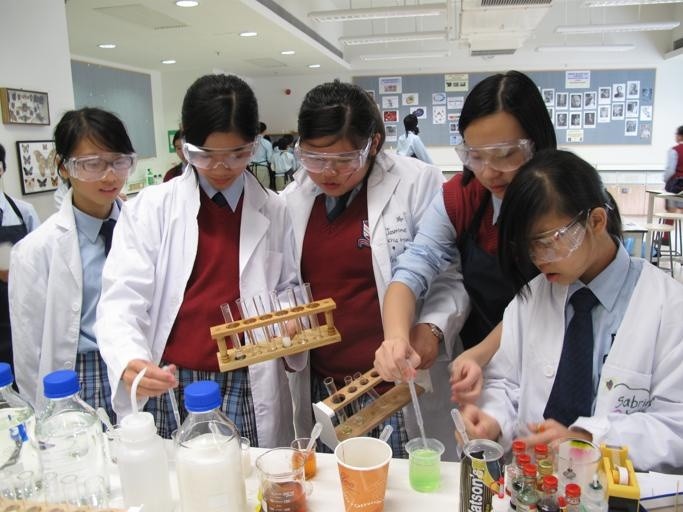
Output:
[335,437,393,512]
[550,438,601,485]
[404,439,444,493]
[255,438,316,512]
[241,436,251,480]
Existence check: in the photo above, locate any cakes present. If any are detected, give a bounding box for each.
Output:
[458,438,508,512]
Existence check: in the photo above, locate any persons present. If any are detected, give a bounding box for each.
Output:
[373,70,558,403]
[8,106,137,432]
[92,73,320,451]
[545,91,553,102]
[246,122,300,192]
[163,130,188,183]
[556,83,638,132]
[279,82,471,458]
[54,183,127,208]
[455,150,682,472]
[394,114,430,162]
[654,125,682,244]
[0,145,41,391]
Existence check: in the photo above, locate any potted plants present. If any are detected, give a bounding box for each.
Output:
[16,139,61,195]
[0,85,51,128]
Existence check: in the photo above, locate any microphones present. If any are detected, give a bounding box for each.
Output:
[652,211,683,267]
[646,224,674,279]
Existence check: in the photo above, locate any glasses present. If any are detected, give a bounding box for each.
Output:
[454,141,536,172]
[61,152,138,182]
[306,0,448,23]
[536,34,634,54]
[180,141,261,170]
[555,7,680,34]
[360,49,448,62]
[339,20,447,45]
[294,142,372,175]
[528,203,609,263]
[66,155,133,172]
[583,1,683,7]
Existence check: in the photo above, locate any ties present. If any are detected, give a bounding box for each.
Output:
[544,288,600,424]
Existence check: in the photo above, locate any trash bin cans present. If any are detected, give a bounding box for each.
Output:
[428,323,445,341]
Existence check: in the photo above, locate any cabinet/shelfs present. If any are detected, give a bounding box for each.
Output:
[489,441,609,512]
[221,283,322,358]
[146,168,162,185]
[0,361,247,512]
[324,372,387,433]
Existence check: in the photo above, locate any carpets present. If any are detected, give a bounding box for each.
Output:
[544,288,600,424]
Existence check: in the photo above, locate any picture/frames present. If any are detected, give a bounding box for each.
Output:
[619,218,649,258]
[645,190,675,224]
